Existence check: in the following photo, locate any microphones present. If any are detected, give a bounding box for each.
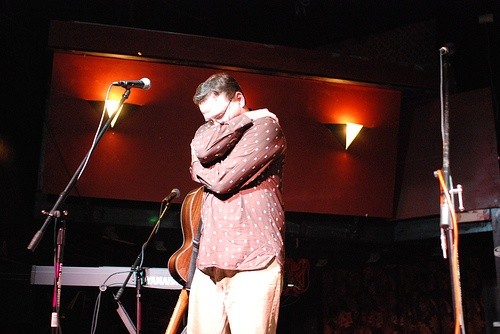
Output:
[162,188,181,203]
[439,47,448,55]
[113,78,151,89]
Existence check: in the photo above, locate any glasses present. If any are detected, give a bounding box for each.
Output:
[205,97,235,126]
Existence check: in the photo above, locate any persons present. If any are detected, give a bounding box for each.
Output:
[181,71,289,334]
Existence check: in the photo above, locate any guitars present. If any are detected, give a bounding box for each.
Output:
[163,183,202,334]
